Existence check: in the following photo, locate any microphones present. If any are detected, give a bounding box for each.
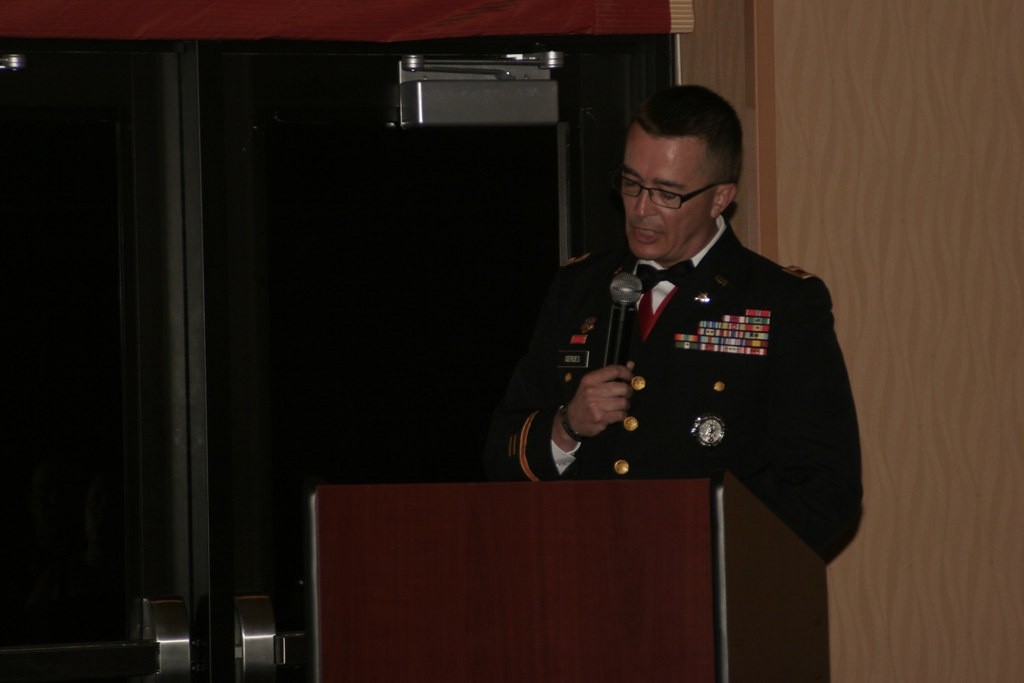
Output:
[601,273,642,383]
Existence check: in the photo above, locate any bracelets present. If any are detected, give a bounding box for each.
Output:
[561,407,585,442]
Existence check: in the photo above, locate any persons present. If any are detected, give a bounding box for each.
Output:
[481,83,863,562]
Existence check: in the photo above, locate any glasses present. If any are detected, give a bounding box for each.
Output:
[608,169,739,209]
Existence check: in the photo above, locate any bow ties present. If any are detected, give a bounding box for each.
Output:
[636,259,696,294]
